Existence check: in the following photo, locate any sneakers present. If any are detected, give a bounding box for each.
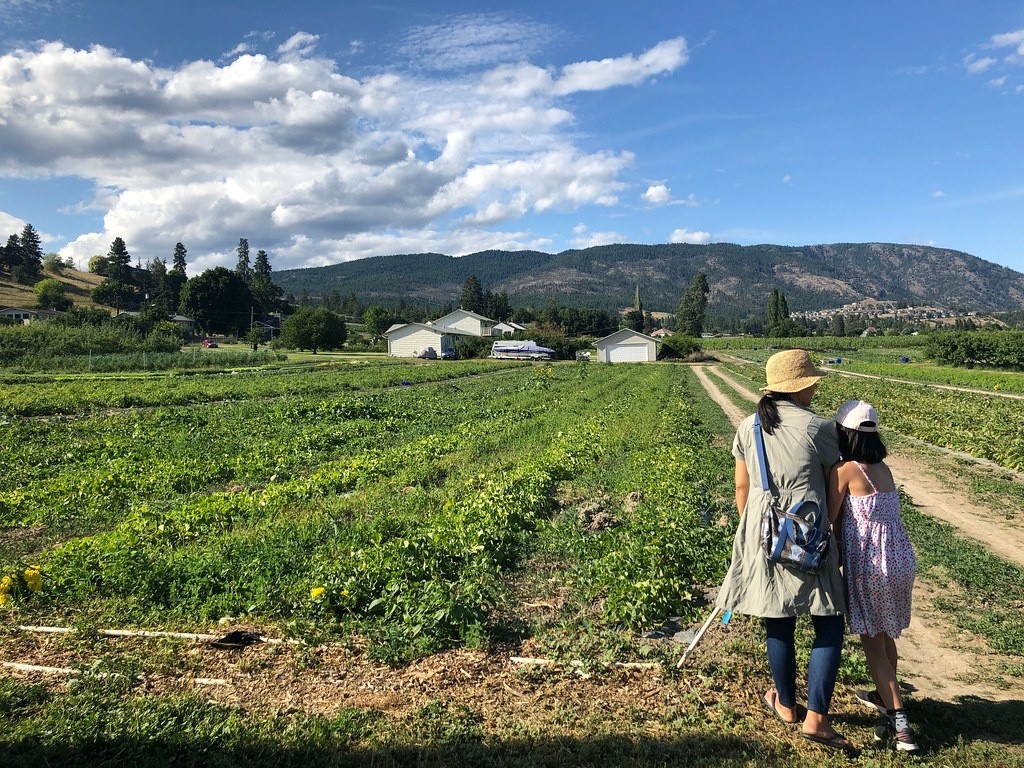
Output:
[855,689,887,714]
[875,722,919,751]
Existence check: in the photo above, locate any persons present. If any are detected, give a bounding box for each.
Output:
[827,401,919,752]
[716,348,851,748]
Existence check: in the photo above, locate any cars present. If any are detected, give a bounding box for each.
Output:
[441,346,457,360]
[202,340,219,348]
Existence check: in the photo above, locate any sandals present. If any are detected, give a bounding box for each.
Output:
[800,733,849,749]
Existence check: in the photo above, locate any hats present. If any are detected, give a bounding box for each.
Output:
[759,349,828,393]
[829,399,878,432]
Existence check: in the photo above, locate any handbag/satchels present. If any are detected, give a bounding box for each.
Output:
[760,499,833,577]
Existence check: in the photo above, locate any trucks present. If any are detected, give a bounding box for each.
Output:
[491,340,553,361]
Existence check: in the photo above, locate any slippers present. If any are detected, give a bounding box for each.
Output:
[762,691,784,720]
